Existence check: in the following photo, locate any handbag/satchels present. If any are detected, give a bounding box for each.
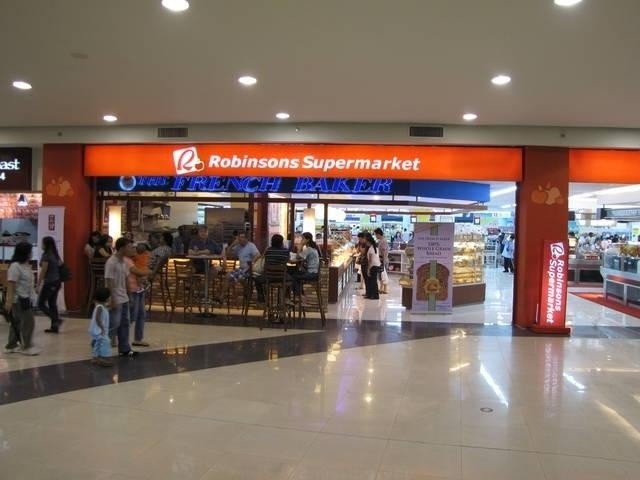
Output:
[21,297,30,309]
[59,263,69,281]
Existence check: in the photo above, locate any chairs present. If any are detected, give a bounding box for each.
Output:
[87,256,326,332]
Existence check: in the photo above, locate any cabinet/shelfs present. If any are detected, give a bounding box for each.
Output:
[453,233,486,305]
[483,238,500,268]
[387,251,408,274]
[599,242,640,306]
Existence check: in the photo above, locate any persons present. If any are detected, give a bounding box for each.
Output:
[493,230,516,273]
[356,228,389,300]
[172,225,322,311]
[83,230,174,367]
[36,236,63,334]
[568,232,626,254]
[4,241,43,355]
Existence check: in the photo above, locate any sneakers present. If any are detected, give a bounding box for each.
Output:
[119,350,137,356]
[4,345,39,356]
